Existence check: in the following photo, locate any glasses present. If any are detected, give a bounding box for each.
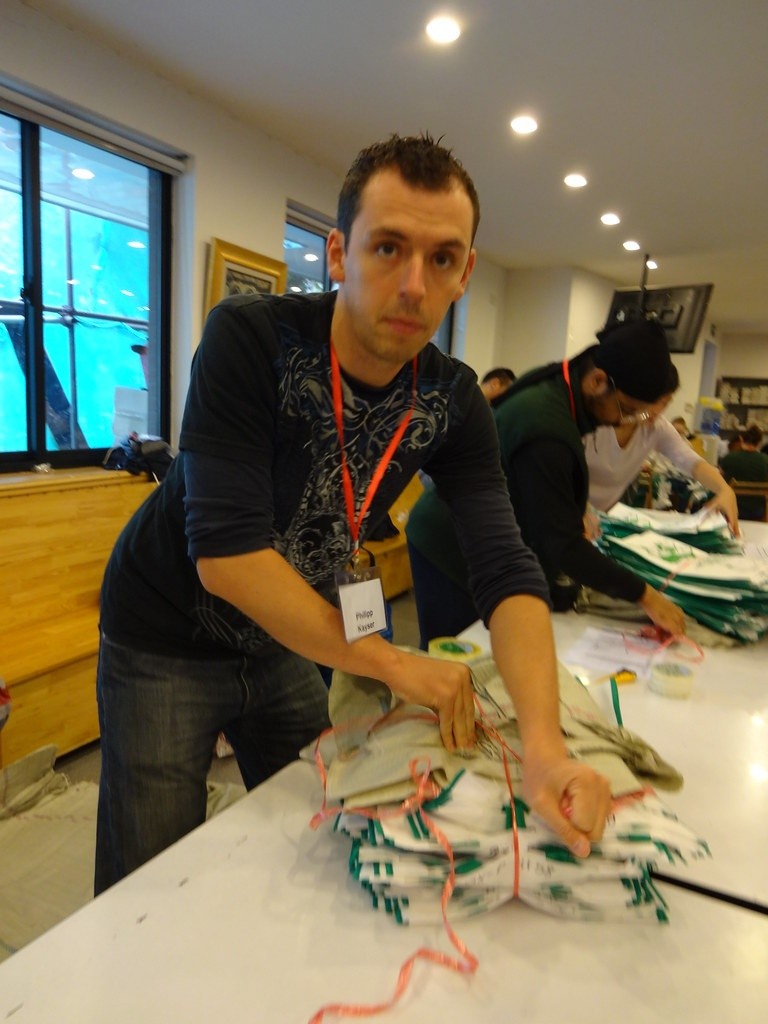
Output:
[608,375,651,426]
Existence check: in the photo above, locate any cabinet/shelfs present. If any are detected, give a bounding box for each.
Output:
[714,376,768,452]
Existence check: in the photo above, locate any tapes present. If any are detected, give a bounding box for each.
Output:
[648,664,696,701]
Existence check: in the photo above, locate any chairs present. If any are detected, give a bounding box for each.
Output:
[729,477,768,521]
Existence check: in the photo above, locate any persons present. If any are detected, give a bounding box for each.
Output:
[94,137,611,900]
[406,317,679,652]
[480,368,516,403]
[697,419,728,468]
[672,417,695,440]
[720,425,768,522]
[582,364,741,541]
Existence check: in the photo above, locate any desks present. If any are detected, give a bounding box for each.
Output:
[0,519,768,1024]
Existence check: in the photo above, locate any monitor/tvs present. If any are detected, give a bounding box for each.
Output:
[596,281,713,354]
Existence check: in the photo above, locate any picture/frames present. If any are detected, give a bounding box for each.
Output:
[203,235,291,329]
